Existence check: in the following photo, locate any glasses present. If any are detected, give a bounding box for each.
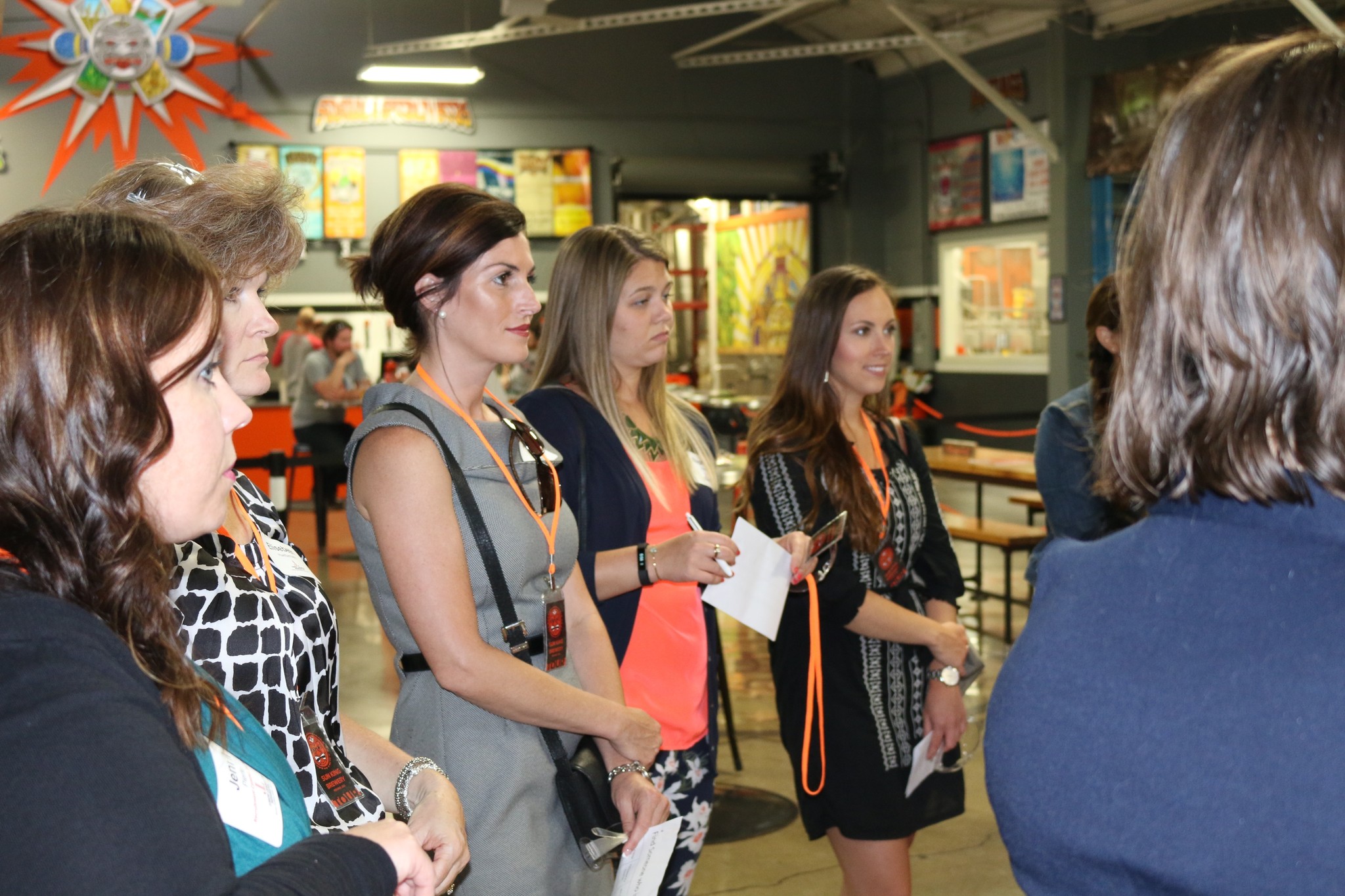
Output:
[511,420,564,514]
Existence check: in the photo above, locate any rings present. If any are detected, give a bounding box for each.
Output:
[713,544,721,559]
[447,883,455,895]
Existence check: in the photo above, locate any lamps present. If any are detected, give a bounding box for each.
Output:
[355,0,490,89]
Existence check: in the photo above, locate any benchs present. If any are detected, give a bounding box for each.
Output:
[1006,480,1057,531]
[933,515,1050,647]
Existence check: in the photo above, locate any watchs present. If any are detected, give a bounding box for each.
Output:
[926,665,962,686]
[607,760,654,784]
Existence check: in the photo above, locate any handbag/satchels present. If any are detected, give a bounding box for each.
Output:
[555,736,626,871]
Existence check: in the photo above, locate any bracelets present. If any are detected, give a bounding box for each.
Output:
[650,545,661,582]
[637,543,653,585]
[394,758,450,821]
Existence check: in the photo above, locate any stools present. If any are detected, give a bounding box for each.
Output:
[283,439,348,552]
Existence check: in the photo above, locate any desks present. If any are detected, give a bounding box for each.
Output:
[916,442,1037,642]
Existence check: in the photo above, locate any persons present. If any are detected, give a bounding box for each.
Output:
[0,158,1160,896]
[982,26,1345,896]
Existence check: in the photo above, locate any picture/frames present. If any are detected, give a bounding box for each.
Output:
[918,114,1061,237]
